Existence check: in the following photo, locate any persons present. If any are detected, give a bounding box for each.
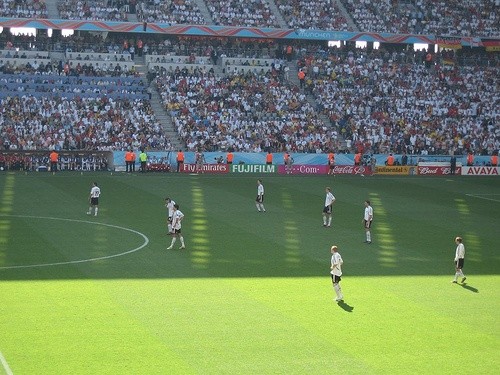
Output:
[361,200,374,244]
[0,0,500,178]
[87,181,101,216]
[166,204,186,250]
[139,149,147,172]
[450,236,466,284]
[322,184,336,227]
[330,246,344,302]
[164,197,177,234]
[128,149,136,172]
[125,150,132,173]
[255,178,266,213]
[176,149,184,173]
[50,149,58,174]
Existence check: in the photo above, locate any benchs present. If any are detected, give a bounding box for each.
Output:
[0,50,290,105]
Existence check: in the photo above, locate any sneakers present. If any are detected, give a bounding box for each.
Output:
[321,224,327,227]
[461,277,466,283]
[333,297,344,302]
[261,210,266,213]
[364,240,372,244]
[93,214,97,216]
[451,280,457,283]
[257,210,261,213]
[325,225,331,228]
[167,246,174,250]
[178,246,185,250]
[87,212,91,215]
